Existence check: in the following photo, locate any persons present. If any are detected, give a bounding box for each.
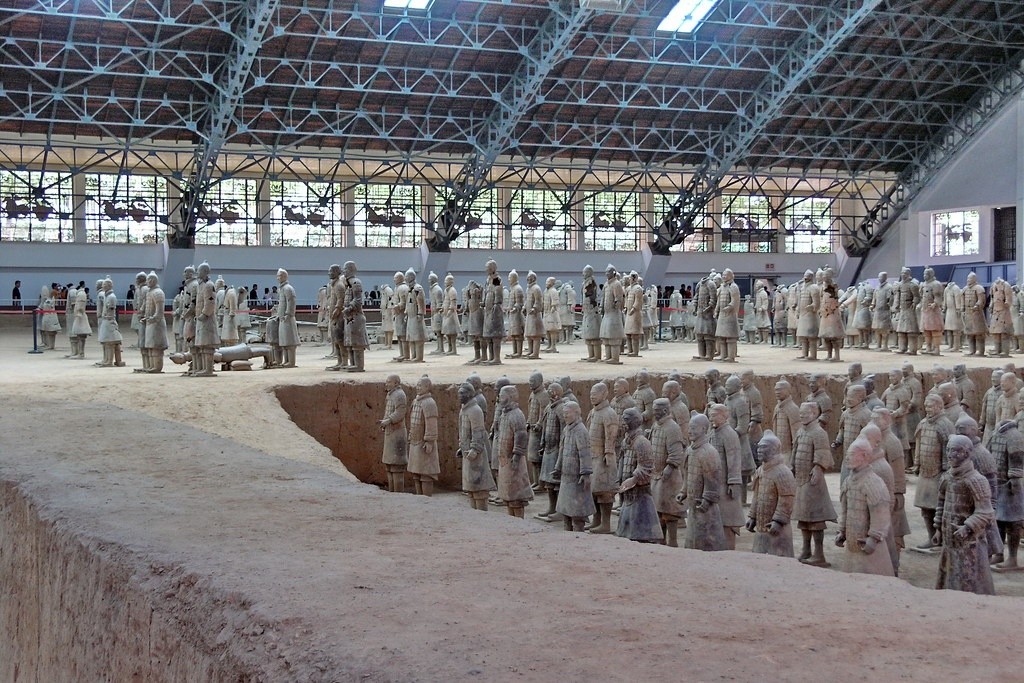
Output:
[380,257,659,366]
[12,280,21,306]
[690,264,1024,362]
[379,365,1024,596]
[96,259,252,377]
[126,285,135,311]
[598,284,604,304]
[169,343,271,369]
[363,291,371,305]
[316,261,370,372]
[684,301,693,343]
[370,286,380,305]
[772,284,781,293]
[657,284,691,307]
[668,290,683,341]
[277,268,301,366]
[265,315,281,365]
[38,286,92,358]
[179,280,185,294]
[243,284,278,310]
[51,280,90,299]
[764,286,770,296]
